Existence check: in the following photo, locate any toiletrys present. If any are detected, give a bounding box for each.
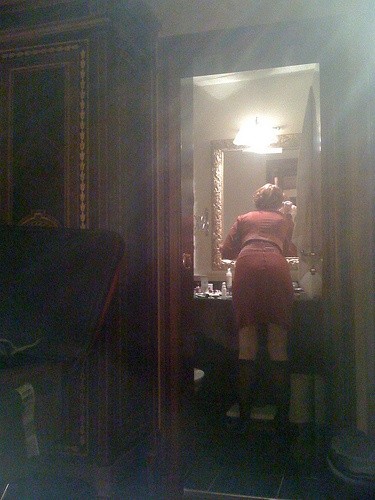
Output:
[291,267,321,304]
[222,282,229,300]
[193,276,222,299]
[225,267,234,289]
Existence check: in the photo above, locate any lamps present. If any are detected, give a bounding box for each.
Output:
[232,116,280,147]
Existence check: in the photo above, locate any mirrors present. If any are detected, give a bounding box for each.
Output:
[210,132,300,271]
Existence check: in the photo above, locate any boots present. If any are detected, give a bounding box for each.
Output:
[266,360,295,438]
[233,355,257,437]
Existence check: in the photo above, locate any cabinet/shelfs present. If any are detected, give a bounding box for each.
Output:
[0,1,161,500]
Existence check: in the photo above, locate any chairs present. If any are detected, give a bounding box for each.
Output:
[1,224,128,485]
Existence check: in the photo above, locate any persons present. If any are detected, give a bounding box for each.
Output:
[220,182,296,449]
